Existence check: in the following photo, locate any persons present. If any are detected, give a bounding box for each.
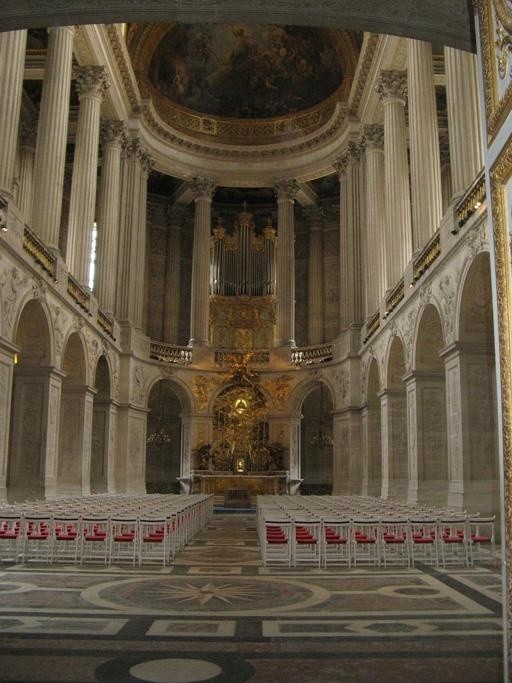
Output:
[245,437,272,470]
[209,437,232,471]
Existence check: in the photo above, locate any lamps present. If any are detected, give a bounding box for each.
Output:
[235,397,248,415]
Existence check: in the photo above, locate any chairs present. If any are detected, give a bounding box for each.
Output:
[0,490,215,568]
[255,492,497,569]
